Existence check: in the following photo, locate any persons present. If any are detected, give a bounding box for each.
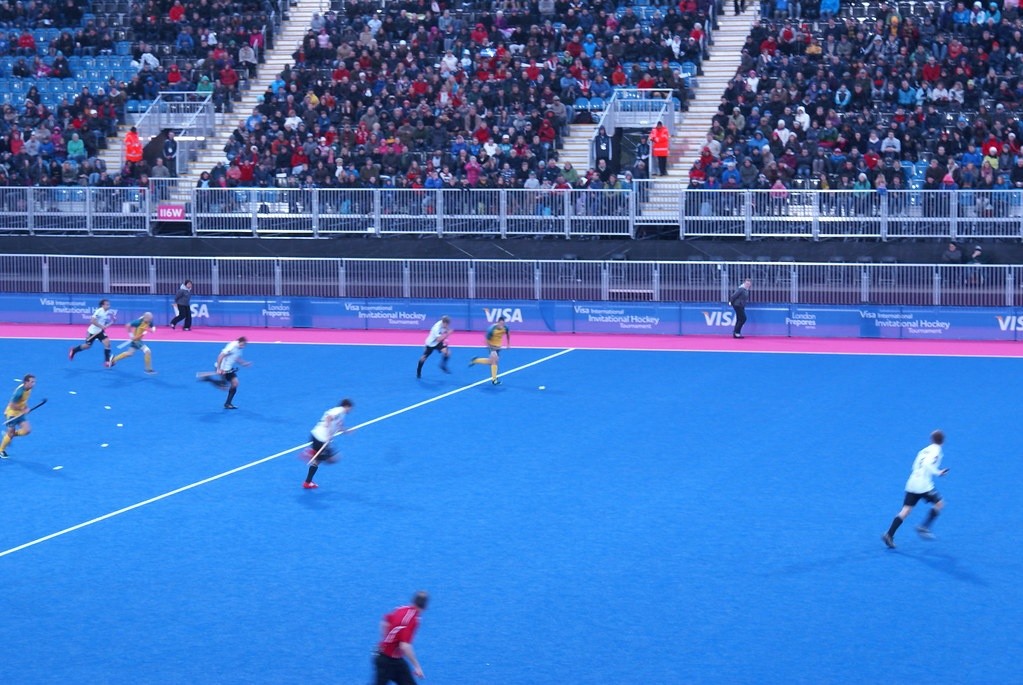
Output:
[728,277,752,339]
[66,298,116,368]
[0,0,1023,284]
[879,430,952,548]
[198,337,253,409]
[369,590,430,685]
[417,315,454,378]
[302,399,354,488]
[171,280,193,332]
[108,312,156,375]
[467,317,512,385]
[0,374,35,458]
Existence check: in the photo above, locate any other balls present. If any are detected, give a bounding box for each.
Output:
[539,386,545,390]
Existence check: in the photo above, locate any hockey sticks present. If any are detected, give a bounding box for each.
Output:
[940,469,949,477]
[307,428,340,466]
[4,398,48,425]
[86,321,114,342]
[196,367,239,377]
[117,330,149,350]
[483,346,507,349]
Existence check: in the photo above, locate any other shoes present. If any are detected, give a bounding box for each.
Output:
[1,430,7,442]
[108,354,115,368]
[68,347,74,361]
[303,481,319,488]
[492,380,501,385]
[183,327,191,331]
[143,369,157,374]
[915,525,931,533]
[733,333,744,339]
[0,451,10,459]
[105,361,110,366]
[468,355,479,368]
[224,403,237,409]
[881,532,896,548]
[170,323,175,329]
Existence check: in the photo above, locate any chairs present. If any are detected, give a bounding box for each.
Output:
[0,14,167,117]
